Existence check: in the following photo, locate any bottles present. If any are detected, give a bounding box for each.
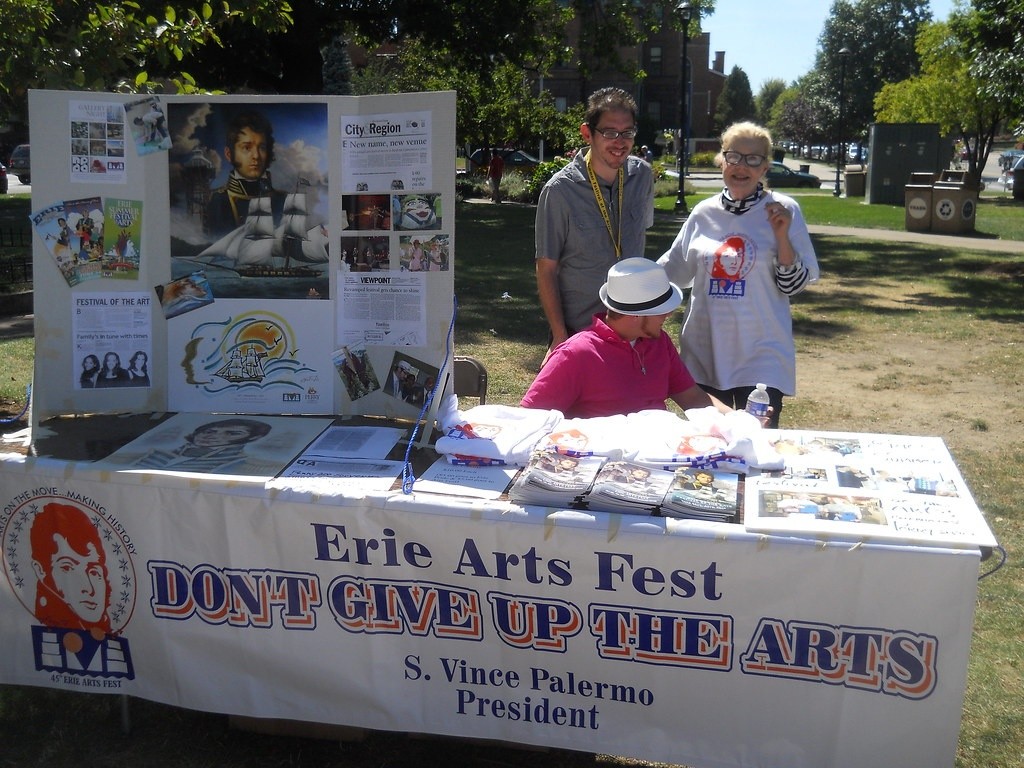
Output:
[745,381,770,428]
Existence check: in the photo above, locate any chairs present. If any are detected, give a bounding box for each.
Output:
[449,354,489,408]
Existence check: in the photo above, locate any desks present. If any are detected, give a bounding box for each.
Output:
[0,398,996,768]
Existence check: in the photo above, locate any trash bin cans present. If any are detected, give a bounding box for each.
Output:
[843,164,864,197]
[932,169,976,234]
[800,165,810,173]
[904,172,935,232]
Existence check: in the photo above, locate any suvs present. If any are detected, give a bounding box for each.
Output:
[469,147,539,179]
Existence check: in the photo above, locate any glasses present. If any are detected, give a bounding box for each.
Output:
[588,126,637,141]
[722,149,767,168]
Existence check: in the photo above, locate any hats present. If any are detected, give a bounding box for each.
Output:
[589,257,684,316]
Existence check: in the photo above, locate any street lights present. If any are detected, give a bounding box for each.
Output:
[674,2,694,216]
[832,47,851,197]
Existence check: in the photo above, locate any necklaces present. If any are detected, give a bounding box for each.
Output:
[631,338,651,376]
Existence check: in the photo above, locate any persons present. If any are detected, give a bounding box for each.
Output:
[641,144,654,163]
[517,257,774,429]
[534,87,655,371]
[487,149,505,205]
[654,120,822,429]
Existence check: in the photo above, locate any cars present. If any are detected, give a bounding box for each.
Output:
[1003,149,1023,156]
[765,162,822,189]
[0,162,8,194]
[780,140,868,163]
[952,145,975,162]
[1005,155,1024,191]
[9,144,30,183]
